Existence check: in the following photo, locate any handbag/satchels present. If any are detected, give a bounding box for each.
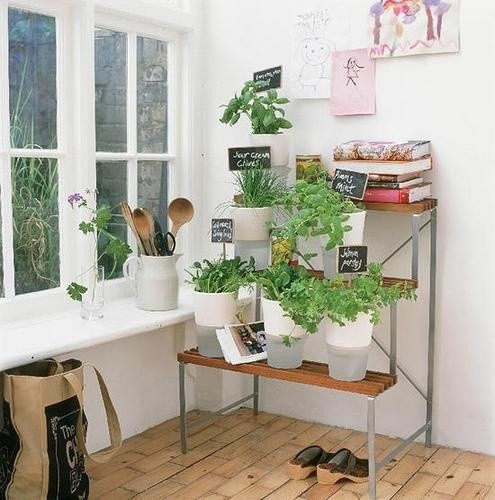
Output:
[0,357,122,500]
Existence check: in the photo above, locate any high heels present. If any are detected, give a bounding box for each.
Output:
[288,445,336,480]
[316,448,369,485]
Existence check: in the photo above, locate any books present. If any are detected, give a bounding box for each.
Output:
[215,321,267,365]
[328,141,432,204]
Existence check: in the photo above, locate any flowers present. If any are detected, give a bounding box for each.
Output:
[65,187,133,304]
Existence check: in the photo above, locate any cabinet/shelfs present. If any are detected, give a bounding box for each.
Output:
[177,194,439,500]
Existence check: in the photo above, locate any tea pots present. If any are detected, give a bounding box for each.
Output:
[122,253,184,310]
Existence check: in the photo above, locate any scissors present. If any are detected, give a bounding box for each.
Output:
[154,231,176,256]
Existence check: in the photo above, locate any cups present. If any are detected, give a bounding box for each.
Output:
[295,153,324,184]
